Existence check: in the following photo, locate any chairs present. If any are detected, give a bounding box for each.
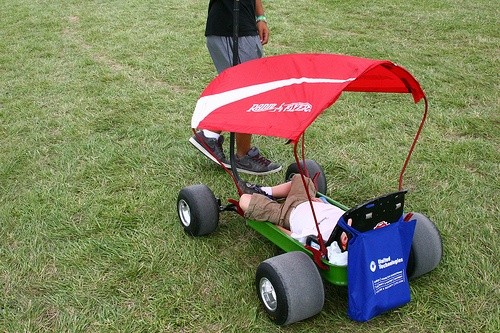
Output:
[306,190,408,261]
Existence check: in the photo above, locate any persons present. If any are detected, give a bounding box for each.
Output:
[189,0,283,175]
[237,174,353,253]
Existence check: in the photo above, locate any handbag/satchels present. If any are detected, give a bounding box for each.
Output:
[338,202,417,325]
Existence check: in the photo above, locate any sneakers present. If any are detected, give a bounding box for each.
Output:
[186,128,232,170]
[237,180,274,201]
[227,146,283,176]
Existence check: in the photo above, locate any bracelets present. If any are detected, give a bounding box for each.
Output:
[255,16,267,22]
